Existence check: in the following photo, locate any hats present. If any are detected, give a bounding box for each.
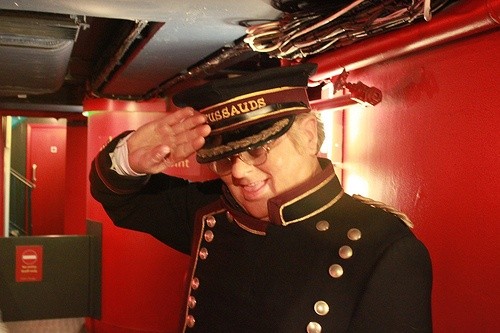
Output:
[174,62,319,163]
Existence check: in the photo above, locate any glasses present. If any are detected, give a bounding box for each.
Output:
[206,136,278,175]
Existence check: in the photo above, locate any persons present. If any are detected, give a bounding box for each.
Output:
[88,62,433,333]
